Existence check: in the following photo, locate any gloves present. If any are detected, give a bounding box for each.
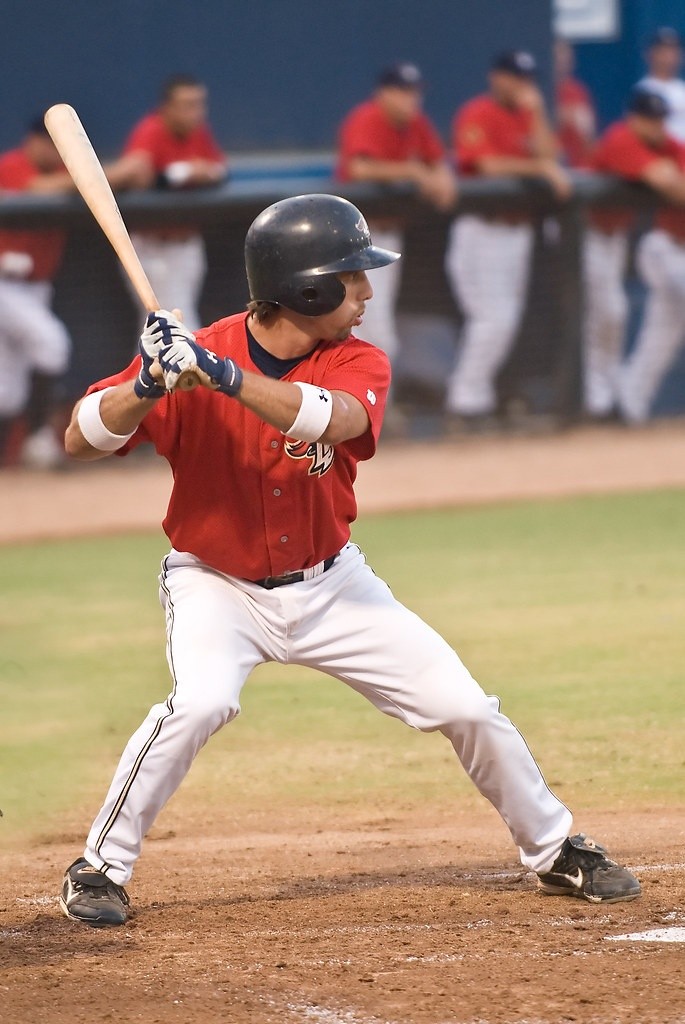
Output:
[135,308,196,401]
[148,339,242,398]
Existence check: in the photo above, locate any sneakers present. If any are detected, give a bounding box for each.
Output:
[57,855,131,927]
[537,834,641,903]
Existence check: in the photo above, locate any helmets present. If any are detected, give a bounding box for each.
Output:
[244,194,400,316]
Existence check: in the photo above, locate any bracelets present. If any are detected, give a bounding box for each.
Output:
[280,380,331,443]
[164,162,188,187]
[78,385,138,452]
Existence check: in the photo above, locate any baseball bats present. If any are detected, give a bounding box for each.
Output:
[45,103,202,394]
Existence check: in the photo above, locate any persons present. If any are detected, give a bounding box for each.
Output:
[0,29,685,467]
[60,193,642,927]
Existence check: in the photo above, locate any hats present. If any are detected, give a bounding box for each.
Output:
[624,91,670,117]
[492,47,538,74]
[382,61,423,88]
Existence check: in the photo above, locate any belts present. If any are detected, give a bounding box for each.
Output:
[251,554,338,589]
